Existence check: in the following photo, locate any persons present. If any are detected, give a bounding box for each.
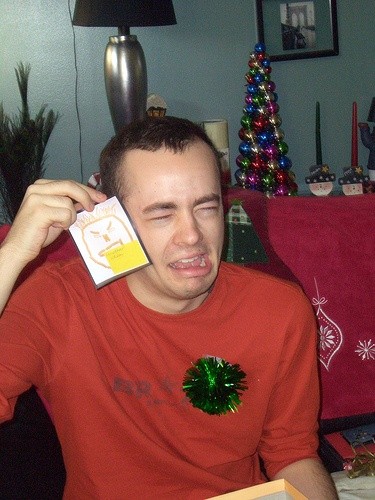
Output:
[0,116,338,500]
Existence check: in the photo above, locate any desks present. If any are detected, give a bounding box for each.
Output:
[221,188,375,419]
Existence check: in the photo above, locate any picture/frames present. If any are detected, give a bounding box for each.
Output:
[255,0,339,63]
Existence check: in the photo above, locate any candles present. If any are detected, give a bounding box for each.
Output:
[351,95,358,167]
[316,101,323,165]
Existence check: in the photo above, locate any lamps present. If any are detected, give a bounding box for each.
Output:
[72,0,178,135]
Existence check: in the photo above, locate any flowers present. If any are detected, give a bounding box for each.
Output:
[342,429,375,479]
